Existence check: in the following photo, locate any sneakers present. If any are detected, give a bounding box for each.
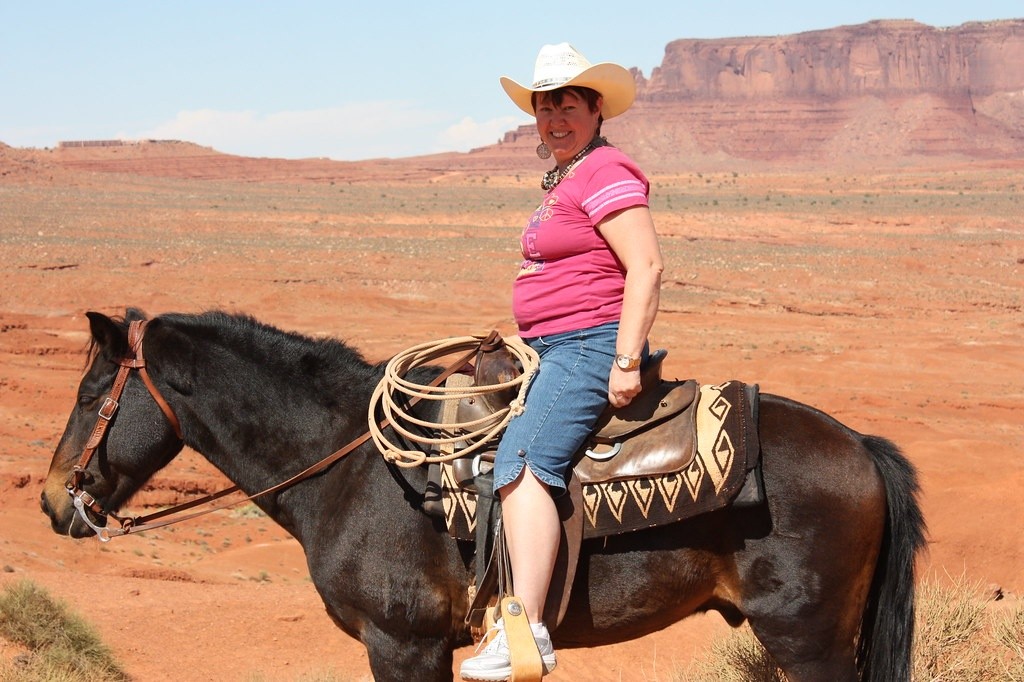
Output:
[460,625,558,682]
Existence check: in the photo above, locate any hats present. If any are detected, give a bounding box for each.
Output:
[500,43,634,122]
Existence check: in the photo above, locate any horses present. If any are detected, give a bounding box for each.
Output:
[39,306,934,682]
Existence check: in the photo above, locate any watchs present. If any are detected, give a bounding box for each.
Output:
[614,353,641,369]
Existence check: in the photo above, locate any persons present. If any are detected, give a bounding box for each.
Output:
[451,44,665,680]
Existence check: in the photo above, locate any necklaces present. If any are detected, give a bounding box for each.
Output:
[540,144,591,190]
